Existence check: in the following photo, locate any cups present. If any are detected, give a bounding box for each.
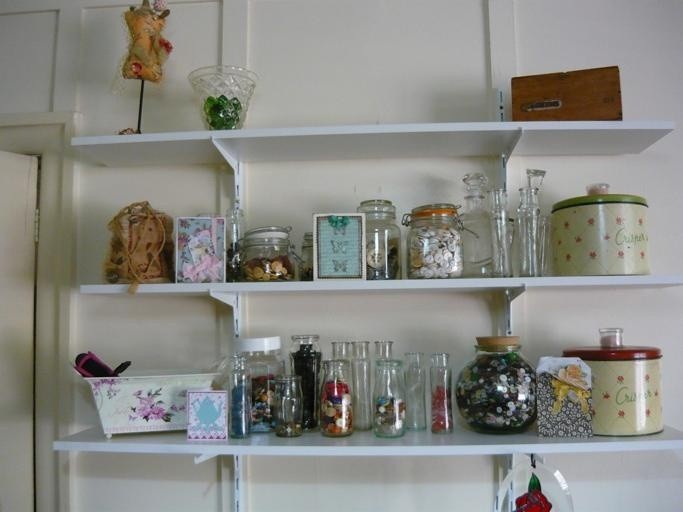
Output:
[587,184,608,195]
[599,328,622,348]
[188,66,259,130]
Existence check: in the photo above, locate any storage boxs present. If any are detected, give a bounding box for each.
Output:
[512,64,623,122]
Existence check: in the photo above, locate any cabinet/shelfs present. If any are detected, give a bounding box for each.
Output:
[49,119,683,461]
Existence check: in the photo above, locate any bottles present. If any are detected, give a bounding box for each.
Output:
[485,188,514,277]
[456,336,537,434]
[298,231,315,280]
[401,203,463,279]
[231,225,298,280]
[357,199,403,280]
[460,181,494,278]
[227,335,452,437]
[516,186,549,277]
